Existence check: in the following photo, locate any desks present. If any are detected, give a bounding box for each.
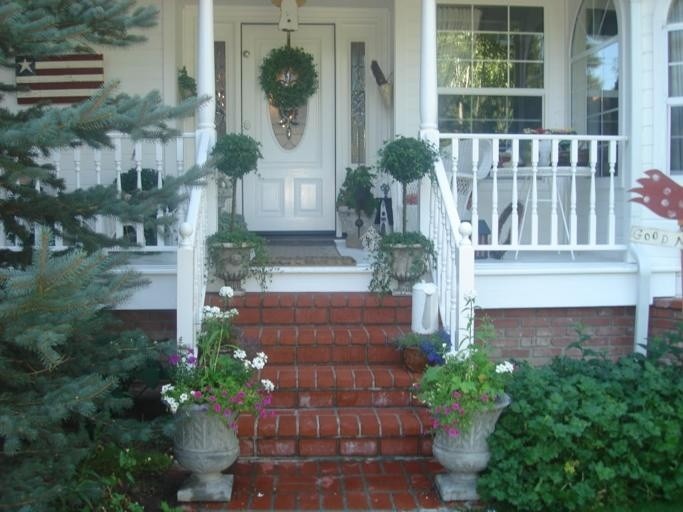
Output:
[491,166,590,262]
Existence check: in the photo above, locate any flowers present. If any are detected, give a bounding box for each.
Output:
[160,285,281,436]
[393,296,506,447]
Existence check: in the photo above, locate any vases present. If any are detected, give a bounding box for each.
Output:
[431,393,510,504]
[170,403,240,503]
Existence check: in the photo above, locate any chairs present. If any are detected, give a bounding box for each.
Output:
[440,138,493,221]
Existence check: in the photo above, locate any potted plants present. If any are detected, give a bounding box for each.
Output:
[203,131,280,299]
[335,134,457,313]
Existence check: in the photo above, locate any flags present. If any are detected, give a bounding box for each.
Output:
[16,54,105,104]
